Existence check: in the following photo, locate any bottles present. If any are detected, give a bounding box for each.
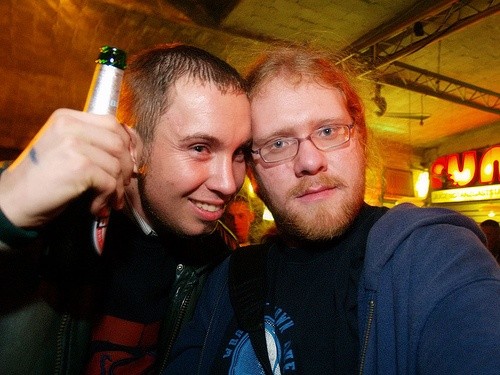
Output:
[24,46,127,320]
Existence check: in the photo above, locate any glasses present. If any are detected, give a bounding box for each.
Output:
[246,116,355,162]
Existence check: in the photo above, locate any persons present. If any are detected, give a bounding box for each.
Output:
[0,44,252,375]
[224,195,257,250]
[163,47,500,375]
